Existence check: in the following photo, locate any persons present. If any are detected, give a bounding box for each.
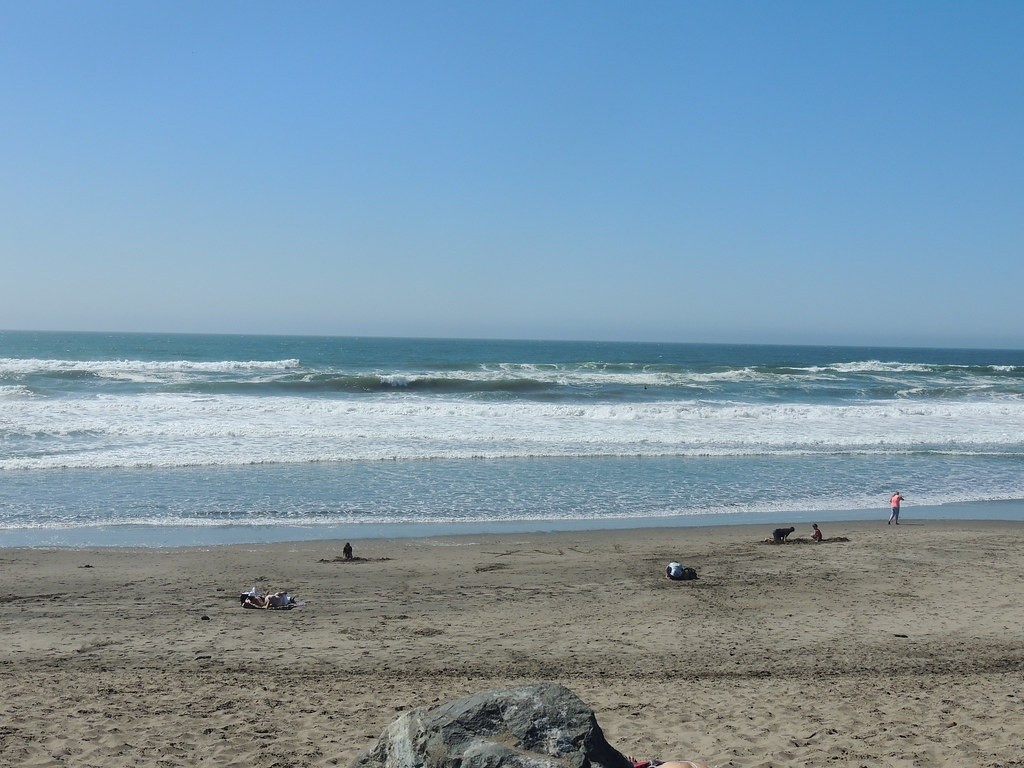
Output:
[810,523,822,542]
[343,542,352,560]
[240,591,296,609]
[888,491,904,525]
[764,527,795,544]
[665,561,683,580]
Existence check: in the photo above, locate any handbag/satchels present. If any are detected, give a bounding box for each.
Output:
[683,567,697,580]
